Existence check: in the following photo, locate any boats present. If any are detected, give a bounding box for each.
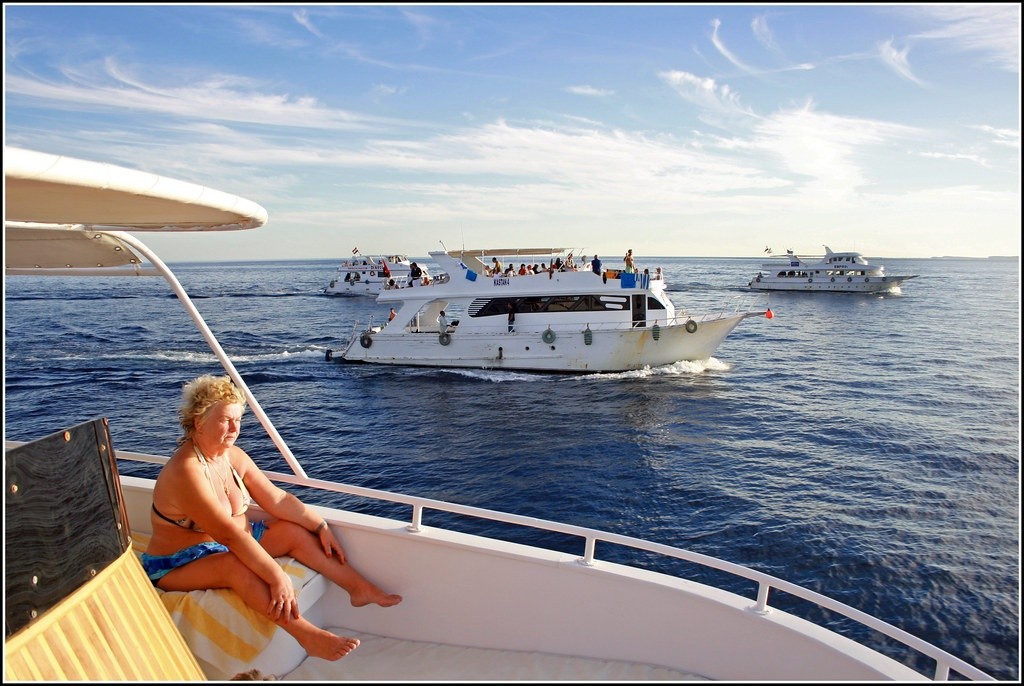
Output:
[313,247,433,296]
[0,144,1005,684]
[324,246,775,373]
[748,244,921,294]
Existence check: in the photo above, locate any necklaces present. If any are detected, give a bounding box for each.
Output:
[204,456,229,496]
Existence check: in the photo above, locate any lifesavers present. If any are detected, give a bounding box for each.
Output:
[686,320,698,333]
[808,279,813,283]
[882,277,887,281]
[329,283,335,288]
[350,279,354,286]
[370,271,375,276]
[360,334,373,348]
[847,277,852,282]
[756,278,761,283]
[542,329,557,344]
[864,278,869,282]
[365,280,369,284]
[439,332,451,346]
[831,277,835,282]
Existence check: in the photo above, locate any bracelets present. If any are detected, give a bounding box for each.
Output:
[316,520,327,532]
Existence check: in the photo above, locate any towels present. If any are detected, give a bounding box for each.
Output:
[620,272,636,288]
[460,262,468,269]
[131,529,318,678]
[465,269,477,282]
[640,273,650,290]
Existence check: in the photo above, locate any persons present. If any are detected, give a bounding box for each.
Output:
[438,311,447,332]
[407,262,422,287]
[656,266,663,280]
[390,308,397,319]
[142,374,402,660]
[624,249,634,273]
[353,274,360,281]
[565,254,573,267]
[485,257,503,277]
[519,263,548,275]
[505,264,515,277]
[507,302,515,332]
[378,260,382,264]
[556,258,565,272]
[592,255,602,275]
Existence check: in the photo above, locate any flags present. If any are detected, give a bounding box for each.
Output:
[352,248,358,254]
